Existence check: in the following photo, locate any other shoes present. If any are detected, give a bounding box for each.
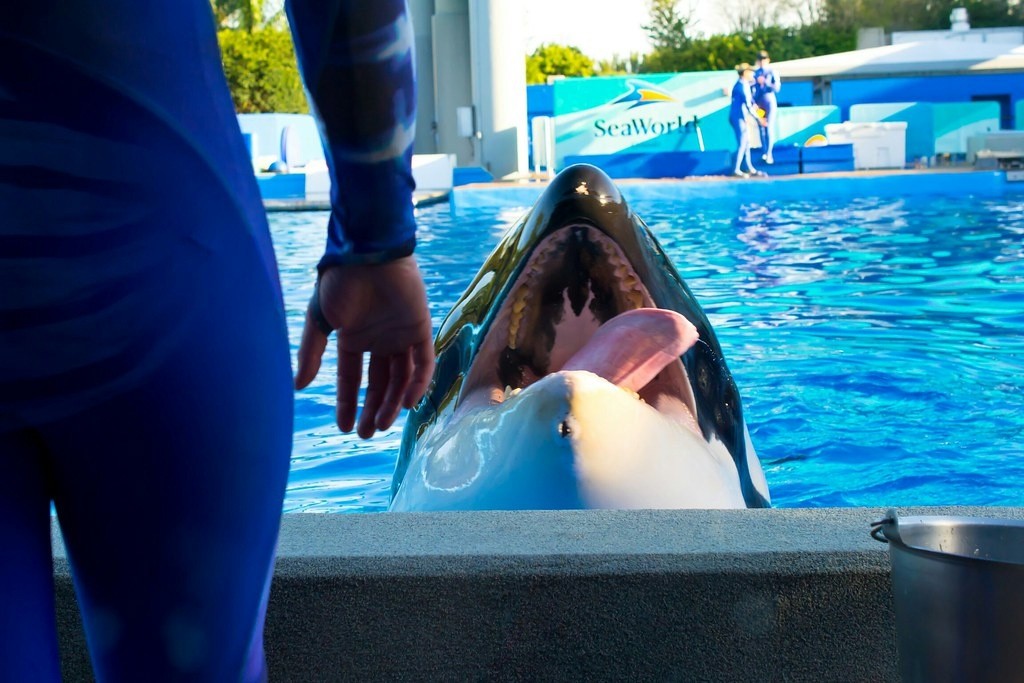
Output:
[751,170,768,177]
[733,171,749,178]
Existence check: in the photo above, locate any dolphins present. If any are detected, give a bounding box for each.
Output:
[383,163,771,509]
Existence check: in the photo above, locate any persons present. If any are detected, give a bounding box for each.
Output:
[729,63,769,177]
[750,51,780,163]
[1,1,437,683]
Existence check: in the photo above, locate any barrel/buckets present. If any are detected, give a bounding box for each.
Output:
[872,509,1023,682]
